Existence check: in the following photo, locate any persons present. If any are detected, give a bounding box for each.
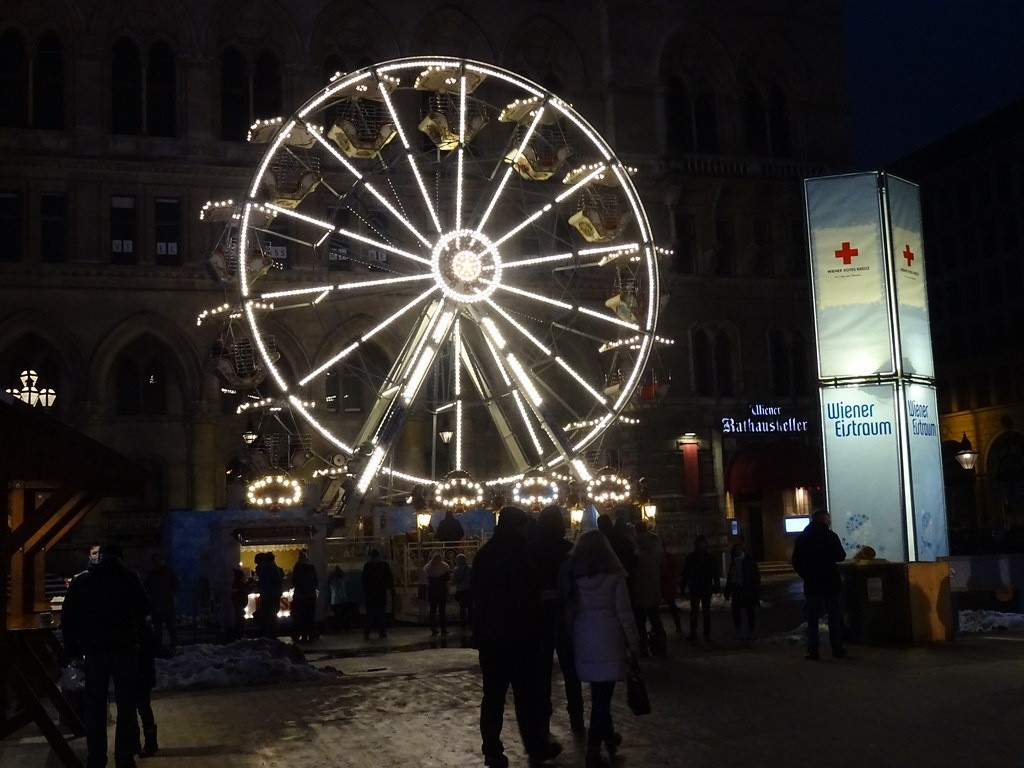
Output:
[723,545,761,640]
[598,509,681,657]
[679,535,720,642]
[362,549,395,640]
[792,509,849,660]
[545,529,643,768]
[471,504,588,768]
[251,552,284,634]
[450,553,474,631]
[435,511,464,547]
[61,537,177,768]
[950,526,1009,556]
[328,564,352,633]
[292,546,319,642]
[423,553,451,636]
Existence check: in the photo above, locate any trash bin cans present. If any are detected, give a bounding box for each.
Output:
[837,561,901,642]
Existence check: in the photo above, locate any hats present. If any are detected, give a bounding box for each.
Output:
[498,507,528,530]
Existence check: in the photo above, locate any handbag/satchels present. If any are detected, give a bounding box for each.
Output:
[419,584,430,599]
[253,605,262,625]
[627,650,651,716]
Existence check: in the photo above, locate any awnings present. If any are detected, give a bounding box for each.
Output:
[726,439,822,500]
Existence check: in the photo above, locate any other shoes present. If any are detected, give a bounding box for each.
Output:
[686,633,697,641]
[705,636,711,642]
[528,742,561,764]
[804,652,819,659]
[485,755,508,765]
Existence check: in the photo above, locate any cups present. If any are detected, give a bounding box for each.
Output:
[39,612,54,628]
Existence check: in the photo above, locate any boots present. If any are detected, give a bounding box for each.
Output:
[585,736,608,768]
[603,715,622,752]
[134,727,142,754]
[139,725,158,758]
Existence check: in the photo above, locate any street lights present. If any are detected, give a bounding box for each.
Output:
[439,424,454,475]
[953,429,986,592]
